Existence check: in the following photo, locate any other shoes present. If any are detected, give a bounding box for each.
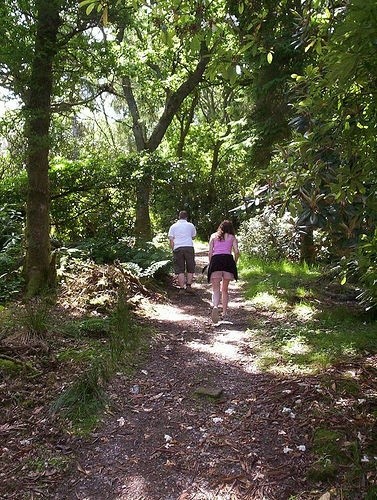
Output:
[187,288,195,293]
[179,289,185,295]
[212,306,219,323]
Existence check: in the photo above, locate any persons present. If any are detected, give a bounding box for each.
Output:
[207,218,239,324]
[168,211,197,293]
